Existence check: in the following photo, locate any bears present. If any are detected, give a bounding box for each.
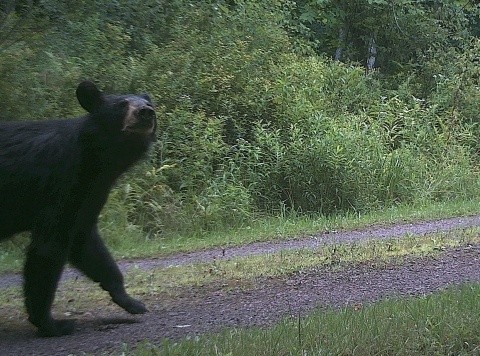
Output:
[0,81,157,338]
